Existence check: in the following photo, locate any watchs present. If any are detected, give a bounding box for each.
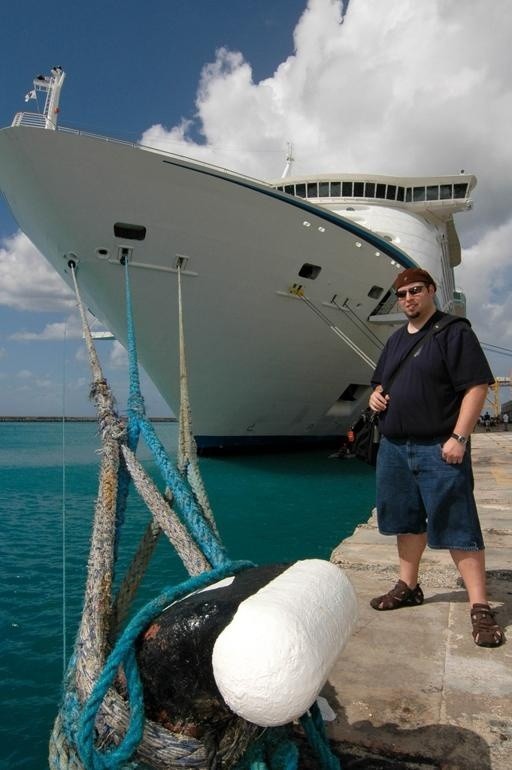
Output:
[450,433,469,445]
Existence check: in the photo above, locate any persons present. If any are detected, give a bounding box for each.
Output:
[475,410,510,433]
[366,266,505,649]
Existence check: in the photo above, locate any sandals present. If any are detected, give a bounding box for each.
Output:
[370,579,423,610]
[471,603,503,648]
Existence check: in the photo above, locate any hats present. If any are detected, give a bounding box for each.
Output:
[395,268,436,292]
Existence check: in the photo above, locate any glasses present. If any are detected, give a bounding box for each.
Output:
[395,286,423,297]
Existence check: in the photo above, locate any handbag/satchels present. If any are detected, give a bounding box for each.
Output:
[348,407,380,458]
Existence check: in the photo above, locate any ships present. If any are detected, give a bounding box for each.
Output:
[0,64,483,459]
